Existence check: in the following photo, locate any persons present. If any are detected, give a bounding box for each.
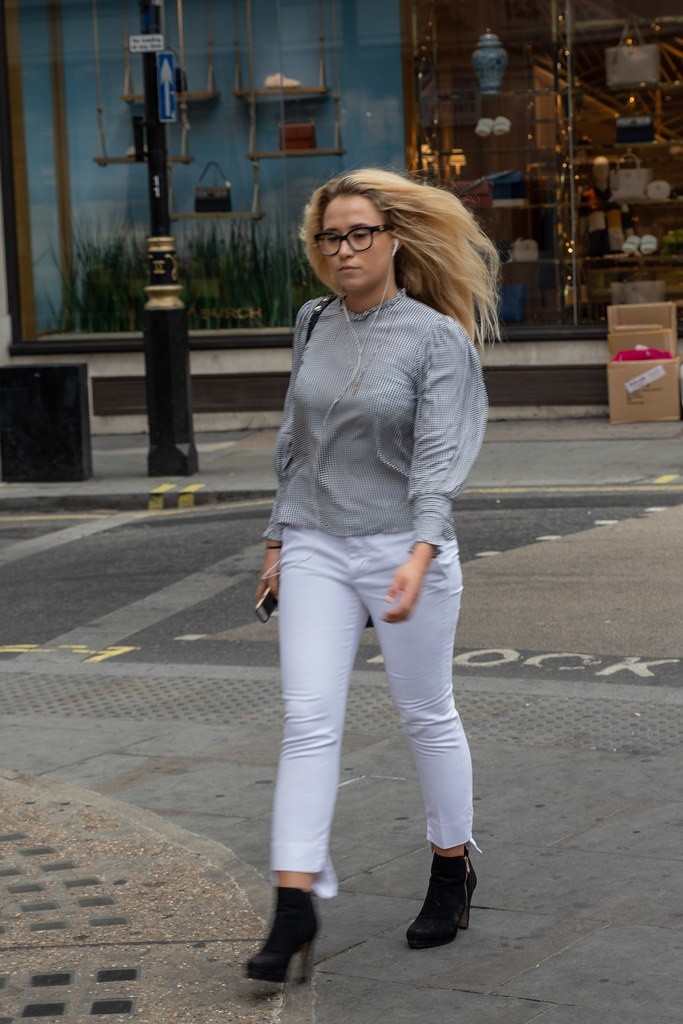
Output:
[244,168,500,982]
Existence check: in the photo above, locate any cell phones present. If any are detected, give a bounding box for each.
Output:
[256,587,278,623]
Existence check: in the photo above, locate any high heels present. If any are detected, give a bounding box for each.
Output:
[406,846,477,950]
[246,886,319,984]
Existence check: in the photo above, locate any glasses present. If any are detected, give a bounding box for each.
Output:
[313,222,395,256]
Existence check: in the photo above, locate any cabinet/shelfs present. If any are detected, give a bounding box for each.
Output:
[411,0,683,322]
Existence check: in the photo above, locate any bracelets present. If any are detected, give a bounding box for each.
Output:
[266,545,280,549]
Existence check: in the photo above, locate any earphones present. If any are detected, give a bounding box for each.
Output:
[391,238,399,257]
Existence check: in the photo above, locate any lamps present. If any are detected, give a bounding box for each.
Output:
[449,148,467,175]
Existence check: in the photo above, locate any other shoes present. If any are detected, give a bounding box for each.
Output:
[621,235,640,252]
[640,235,658,255]
[265,73,301,87]
[475,117,493,137]
[493,116,510,134]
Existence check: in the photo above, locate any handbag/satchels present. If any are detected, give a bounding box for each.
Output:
[449,178,492,208]
[508,238,540,263]
[491,169,532,208]
[278,121,316,150]
[493,282,527,324]
[166,46,187,94]
[193,161,233,212]
[608,152,652,201]
[493,243,510,262]
[614,114,654,143]
[605,14,661,87]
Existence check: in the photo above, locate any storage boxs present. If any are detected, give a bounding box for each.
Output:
[495,284,526,322]
[607,301,683,424]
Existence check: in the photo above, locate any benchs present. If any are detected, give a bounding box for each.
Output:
[587,267,683,320]
